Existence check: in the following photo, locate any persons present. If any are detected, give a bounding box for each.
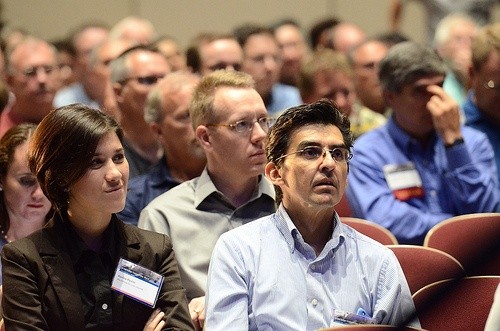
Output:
[135,70,284,325]
[0,104,199,331]
[202,95,426,331]
[0,12,499,243]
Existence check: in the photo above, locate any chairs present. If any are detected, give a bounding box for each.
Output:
[411,275,500,331]
[385,244,464,296]
[424,213,500,276]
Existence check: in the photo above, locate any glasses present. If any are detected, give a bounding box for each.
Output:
[274,146,353,163]
[207,59,245,72]
[115,73,169,85]
[246,52,289,63]
[199,116,278,132]
